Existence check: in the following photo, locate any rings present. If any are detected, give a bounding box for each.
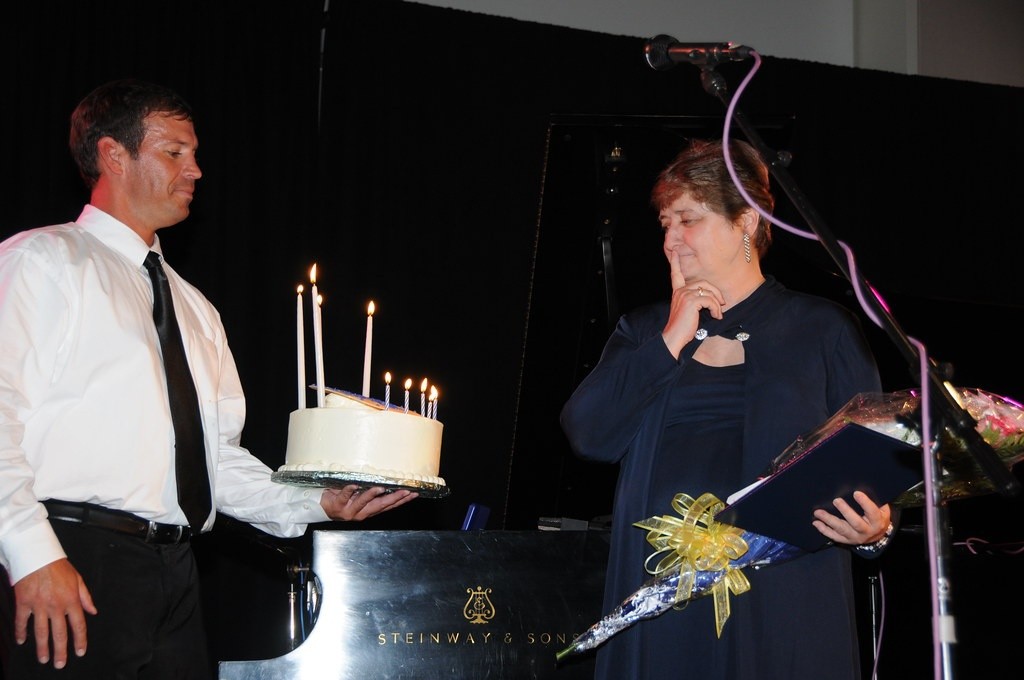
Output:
[697,286,704,296]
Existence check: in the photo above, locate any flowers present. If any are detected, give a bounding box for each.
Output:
[557,382,1024,661]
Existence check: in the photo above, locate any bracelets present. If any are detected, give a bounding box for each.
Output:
[856,522,894,553]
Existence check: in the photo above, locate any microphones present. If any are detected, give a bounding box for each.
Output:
[644,34,754,71]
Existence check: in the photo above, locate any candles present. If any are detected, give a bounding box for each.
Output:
[308,262,327,409]
[428,394,434,417]
[404,378,412,414]
[297,285,306,409]
[384,371,392,409]
[430,385,438,420]
[420,377,427,416]
[362,300,376,397]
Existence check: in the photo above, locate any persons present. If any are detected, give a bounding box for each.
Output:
[558,138,896,680]
[0,76,419,680]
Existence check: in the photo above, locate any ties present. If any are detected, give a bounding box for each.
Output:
[143,251,212,535]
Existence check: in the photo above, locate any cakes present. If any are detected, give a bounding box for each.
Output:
[278,383,448,486]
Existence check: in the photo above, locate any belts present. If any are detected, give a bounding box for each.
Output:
[41,502,192,545]
